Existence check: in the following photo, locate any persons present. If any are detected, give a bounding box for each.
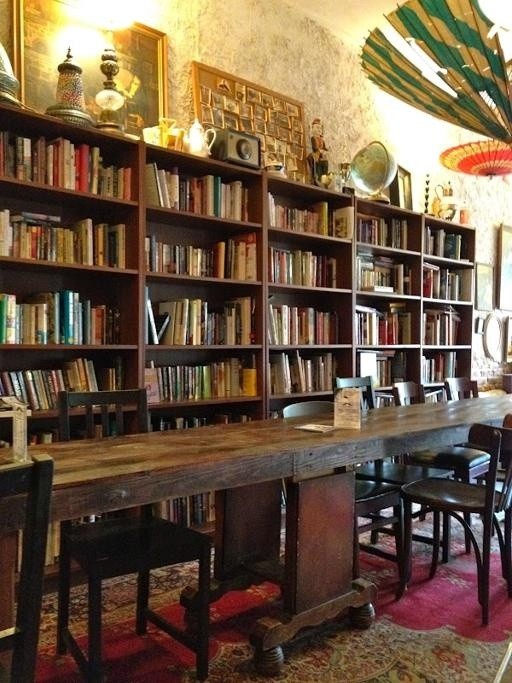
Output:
[306,120,328,188]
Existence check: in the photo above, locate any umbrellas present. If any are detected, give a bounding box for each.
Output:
[440,140,512,180]
[358,0,512,142]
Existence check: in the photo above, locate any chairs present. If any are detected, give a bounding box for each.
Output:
[400,429,511,627]
[331,376,456,565]
[277,400,405,591]
[445,376,511,520]
[52,387,211,680]
[392,382,495,555]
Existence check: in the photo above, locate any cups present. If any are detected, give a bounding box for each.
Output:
[459,207,470,224]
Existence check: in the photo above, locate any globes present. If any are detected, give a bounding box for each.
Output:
[351,141,398,204]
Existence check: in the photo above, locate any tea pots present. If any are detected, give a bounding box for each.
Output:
[436,180,458,219]
[186,117,216,158]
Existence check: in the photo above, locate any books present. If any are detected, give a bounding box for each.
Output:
[144,161,260,432]
[422,226,463,391]
[172,490,217,532]
[355,215,415,407]
[266,192,353,420]
[1,128,132,446]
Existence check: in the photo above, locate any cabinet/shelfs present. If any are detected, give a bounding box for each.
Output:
[0,101,479,601]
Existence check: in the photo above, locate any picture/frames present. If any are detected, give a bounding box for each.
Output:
[8,0,173,142]
[492,220,511,314]
[471,261,495,314]
[501,311,511,364]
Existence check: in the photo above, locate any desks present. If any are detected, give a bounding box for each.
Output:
[0,398,510,683]
